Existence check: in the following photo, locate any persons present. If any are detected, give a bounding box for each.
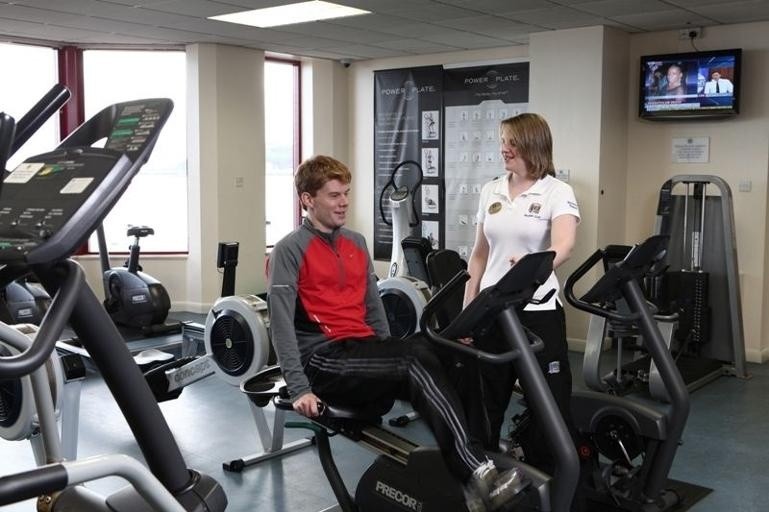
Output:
[266,157,528,504]
[651,62,733,104]
[459,95,577,456]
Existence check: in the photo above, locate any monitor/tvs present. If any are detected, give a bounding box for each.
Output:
[639,48,741,120]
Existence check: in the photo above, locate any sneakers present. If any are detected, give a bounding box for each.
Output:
[471,460,522,503]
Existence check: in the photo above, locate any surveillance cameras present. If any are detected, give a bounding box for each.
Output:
[340,59,352,68]
